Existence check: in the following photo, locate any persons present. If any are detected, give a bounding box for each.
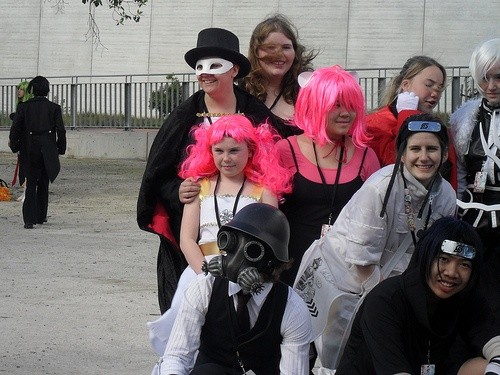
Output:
[137,16,500,375]
[7,76,67,229]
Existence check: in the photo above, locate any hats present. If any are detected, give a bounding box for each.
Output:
[184,28,251,80]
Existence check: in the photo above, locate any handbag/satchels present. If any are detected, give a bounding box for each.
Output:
[0,179,11,201]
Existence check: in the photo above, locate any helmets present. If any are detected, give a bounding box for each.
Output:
[216,203,290,263]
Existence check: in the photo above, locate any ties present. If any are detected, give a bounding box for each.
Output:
[235,289,252,339]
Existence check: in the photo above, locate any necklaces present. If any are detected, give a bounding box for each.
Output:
[268,87,285,111]
[203,97,238,126]
[214,171,246,228]
[400,162,432,249]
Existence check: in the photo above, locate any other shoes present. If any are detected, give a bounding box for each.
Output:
[24,222,33,229]
